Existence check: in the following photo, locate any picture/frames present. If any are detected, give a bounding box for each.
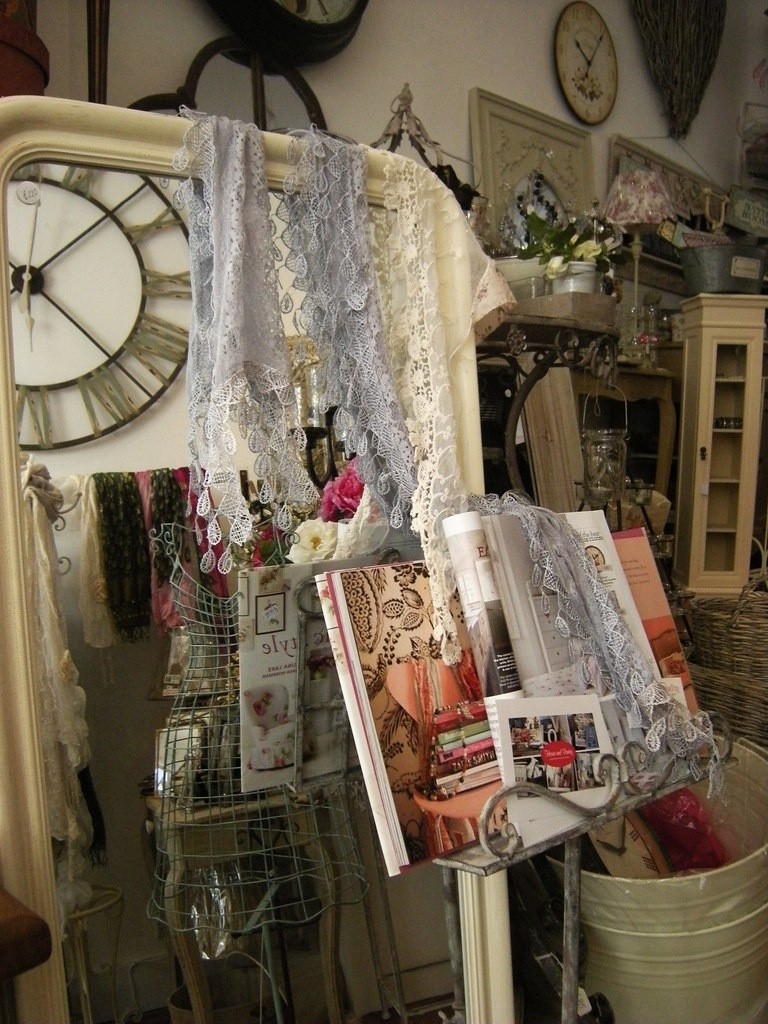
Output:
[470,87,597,252]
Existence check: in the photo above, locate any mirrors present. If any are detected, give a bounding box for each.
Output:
[0,95,516,1024]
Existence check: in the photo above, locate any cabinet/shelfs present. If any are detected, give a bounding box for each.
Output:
[621,293,768,637]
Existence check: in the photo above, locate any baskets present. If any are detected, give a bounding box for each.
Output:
[686,576,767,680]
[686,661,768,749]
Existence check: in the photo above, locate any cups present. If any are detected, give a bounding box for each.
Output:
[670,312,685,344]
[618,304,658,346]
[663,583,684,597]
[647,533,674,557]
[627,483,654,506]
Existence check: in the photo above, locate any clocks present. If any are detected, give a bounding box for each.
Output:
[554,0,618,124]
[3,163,193,452]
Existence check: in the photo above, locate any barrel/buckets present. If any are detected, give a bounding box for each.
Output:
[674,243,767,297]
[545,734,768,1024]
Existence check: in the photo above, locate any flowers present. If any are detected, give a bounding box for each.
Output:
[518,211,627,296]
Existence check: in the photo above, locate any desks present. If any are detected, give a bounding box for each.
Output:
[475,353,676,528]
[475,293,621,506]
[145,792,341,1024]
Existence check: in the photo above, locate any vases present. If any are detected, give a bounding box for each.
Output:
[551,261,598,294]
